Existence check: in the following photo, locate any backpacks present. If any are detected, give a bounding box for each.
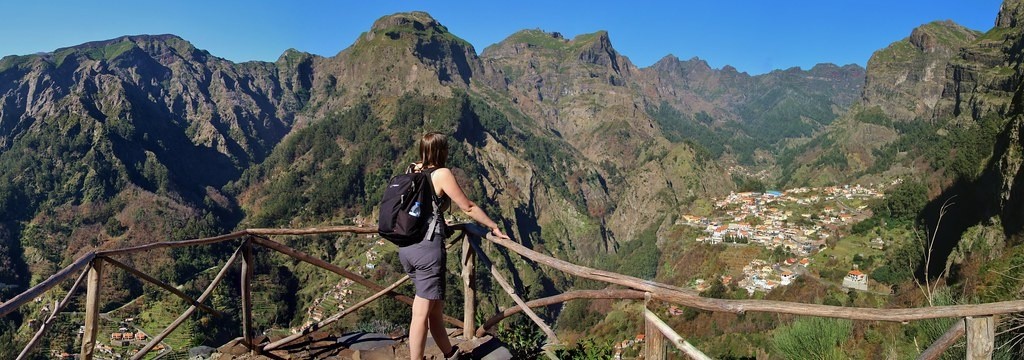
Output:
[378,161,448,247]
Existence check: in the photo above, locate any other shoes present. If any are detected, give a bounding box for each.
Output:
[444,346,474,360]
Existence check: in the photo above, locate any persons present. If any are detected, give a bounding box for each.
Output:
[398,131,510,360]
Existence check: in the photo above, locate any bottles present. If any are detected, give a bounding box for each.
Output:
[409,202,420,217]
